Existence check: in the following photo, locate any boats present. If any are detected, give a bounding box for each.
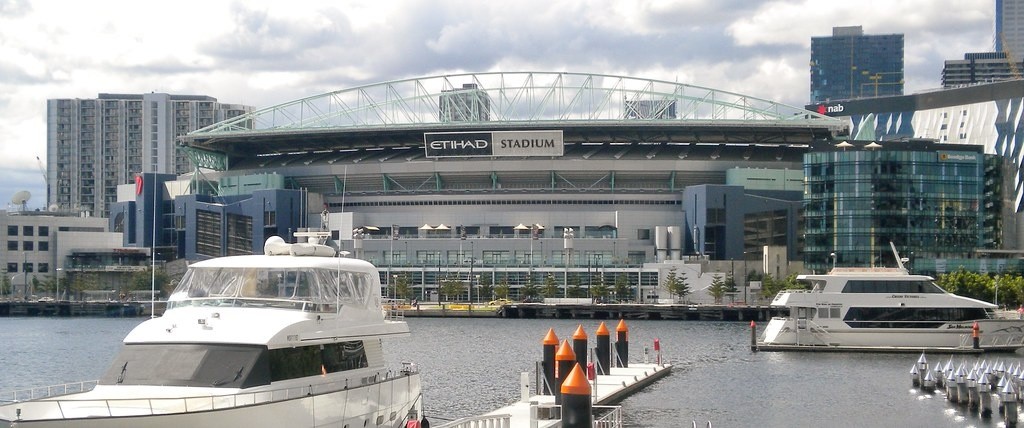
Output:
[754,240,1024,348]
[0,165,429,428]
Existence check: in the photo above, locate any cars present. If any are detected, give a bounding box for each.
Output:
[523,294,544,304]
[489,298,512,307]
[27,293,55,303]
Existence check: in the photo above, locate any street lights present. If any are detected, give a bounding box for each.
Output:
[55,267,63,301]
[529,224,538,285]
